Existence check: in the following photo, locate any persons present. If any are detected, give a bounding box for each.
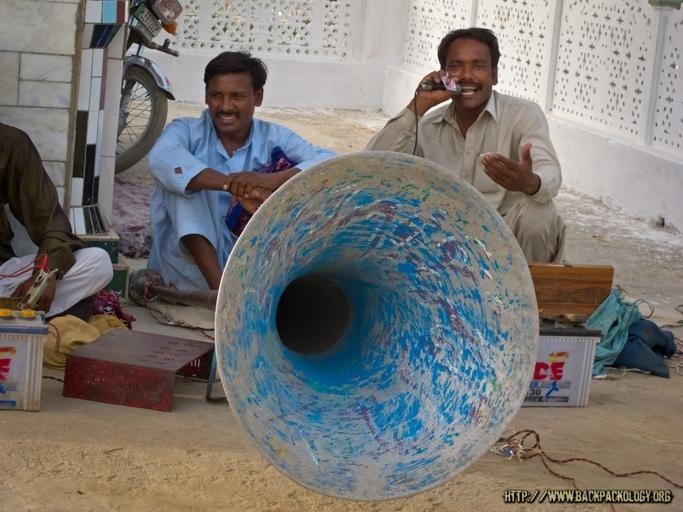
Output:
[363,28,566,264]
[145,51,339,292]
[0,122,113,320]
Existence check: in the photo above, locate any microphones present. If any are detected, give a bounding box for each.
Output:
[421,78,462,96]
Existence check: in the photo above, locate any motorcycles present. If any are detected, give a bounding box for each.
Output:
[112,0,183,175]
